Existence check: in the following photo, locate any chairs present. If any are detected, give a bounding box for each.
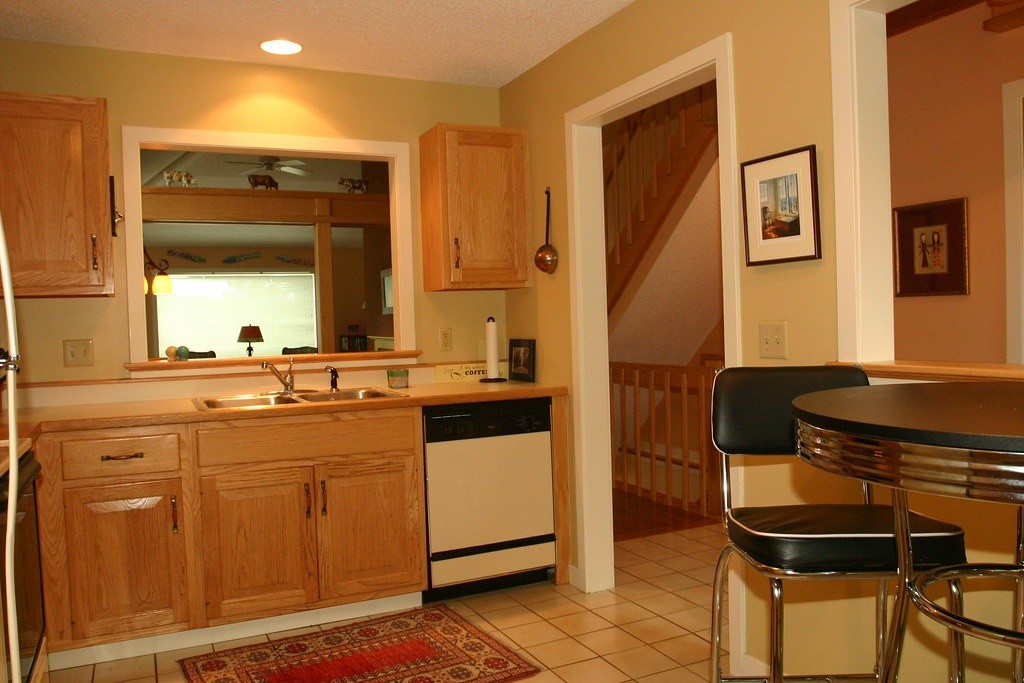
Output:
[711,364,967,683]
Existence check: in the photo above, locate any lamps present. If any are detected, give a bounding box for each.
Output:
[238,326,264,359]
[143,248,172,295]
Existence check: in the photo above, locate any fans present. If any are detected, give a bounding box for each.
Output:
[226,152,310,175]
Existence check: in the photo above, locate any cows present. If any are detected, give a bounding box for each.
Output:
[248,175,278,189]
[163,170,197,188]
[339,176,369,193]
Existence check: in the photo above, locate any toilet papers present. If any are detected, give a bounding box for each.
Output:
[484,322,500,379]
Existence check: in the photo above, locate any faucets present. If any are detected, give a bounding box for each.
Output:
[261,357,295,390]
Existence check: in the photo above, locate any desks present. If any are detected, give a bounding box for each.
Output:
[367,335,394,351]
[790,379,1024,682]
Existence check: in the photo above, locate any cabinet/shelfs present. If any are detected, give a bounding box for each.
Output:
[422,122,528,293]
[47,428,192,640]
[0,91,117,299]
[197,407,425,627]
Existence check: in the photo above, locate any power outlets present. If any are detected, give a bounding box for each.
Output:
[63,336,94,369]
[439,327,451,351]
[758,321,789,359]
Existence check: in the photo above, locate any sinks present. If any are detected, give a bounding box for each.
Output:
[295,387,410,404]
[190,395,301,410]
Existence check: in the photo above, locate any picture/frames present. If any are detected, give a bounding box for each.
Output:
[893,196,969,297]
[740,146,816,264]
[508,338,536,384]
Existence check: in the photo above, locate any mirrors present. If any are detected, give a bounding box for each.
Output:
[382,269,395,314]
[122,129,423,368]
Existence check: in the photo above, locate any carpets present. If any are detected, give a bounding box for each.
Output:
[175,602,540,682]
